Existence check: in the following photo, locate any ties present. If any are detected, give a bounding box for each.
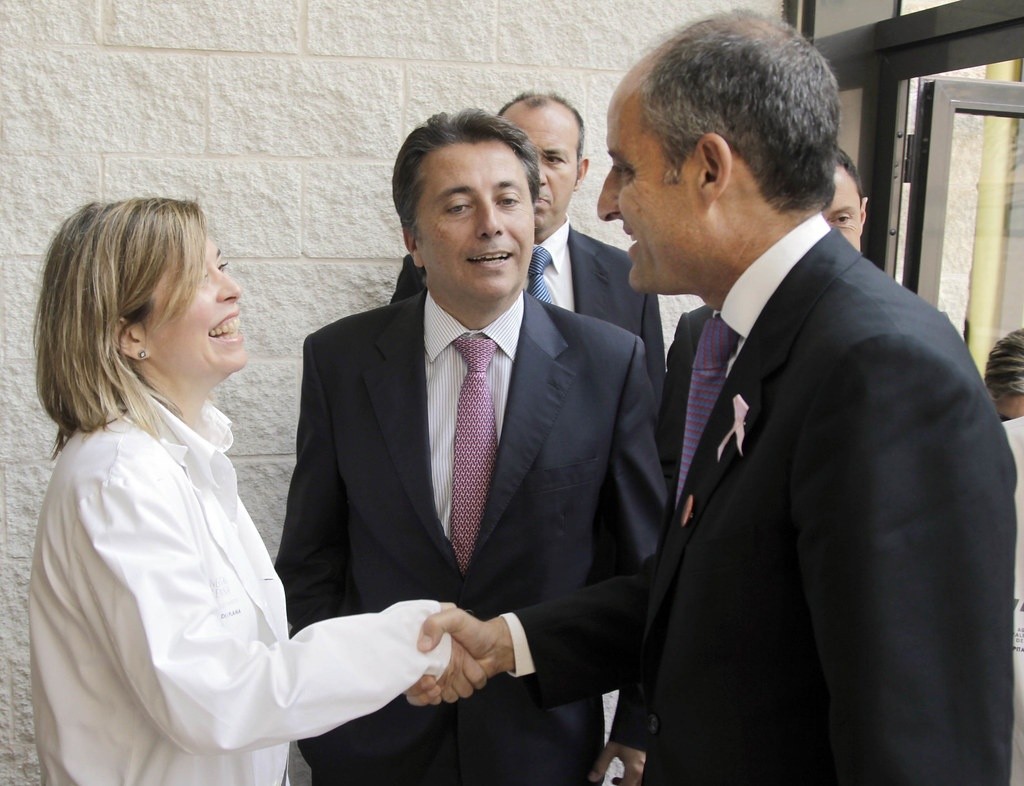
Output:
[675,313,739,510]
[451,337,498,575]
[526,246,552,303]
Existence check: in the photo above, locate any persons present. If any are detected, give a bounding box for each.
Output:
[28,197,486,786]
[595,10,1016,786]
[656,147,868,506]
[273,111,670,786]
[394,90,665,415]
[984,328,1024,786]
